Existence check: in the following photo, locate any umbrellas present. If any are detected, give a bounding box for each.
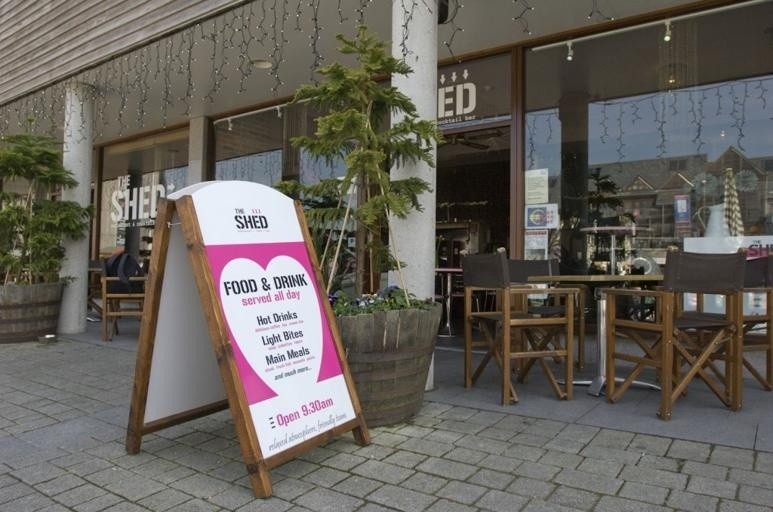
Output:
[723,168,744,252]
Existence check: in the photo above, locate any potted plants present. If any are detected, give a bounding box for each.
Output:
[0,116,98,344]
[269,21,443,429]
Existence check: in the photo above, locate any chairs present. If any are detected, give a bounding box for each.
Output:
[459,248,578,406]
[99,258,147,341]
[600,244,747,421]
[697,248,773,394]
[89,261,103,313]
[495,259,587,383]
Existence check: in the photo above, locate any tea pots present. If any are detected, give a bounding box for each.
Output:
[696,202,731,239]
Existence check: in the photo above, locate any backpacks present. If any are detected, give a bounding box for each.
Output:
[106,251,144,294]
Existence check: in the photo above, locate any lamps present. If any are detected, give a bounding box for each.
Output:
[227,120,233,131]
[530,0,773,62]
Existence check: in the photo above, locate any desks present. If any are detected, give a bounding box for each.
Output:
[437,268,464,338]
[525,275,663,397]
